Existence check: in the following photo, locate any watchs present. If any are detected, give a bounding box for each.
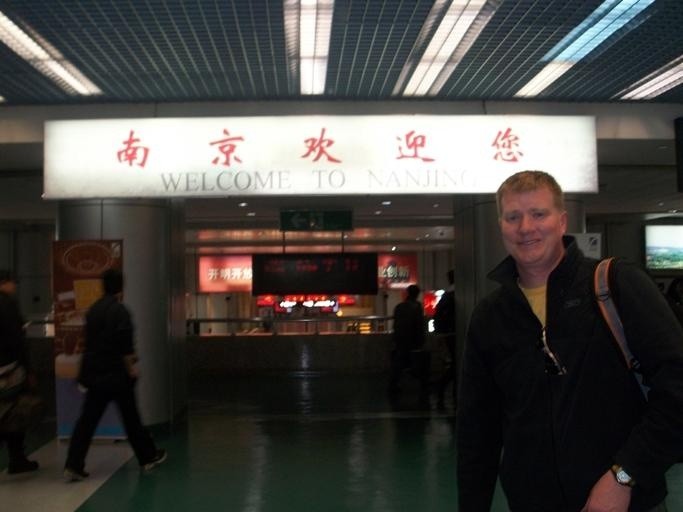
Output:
[610,464,637,489]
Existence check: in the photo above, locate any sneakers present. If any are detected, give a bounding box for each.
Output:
[143,449,167,470]
[8,458,40,475]
[63,461,90,481]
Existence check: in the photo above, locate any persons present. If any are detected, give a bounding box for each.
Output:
[434,270,455,410]
[0,270,38,474]
[63,269,167,481]
[457,171,683,512]
[388,285,431,407]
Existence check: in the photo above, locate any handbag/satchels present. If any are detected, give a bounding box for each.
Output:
[1,362,24,388]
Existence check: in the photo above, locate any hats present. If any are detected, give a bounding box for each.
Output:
[104,269,124,294]
